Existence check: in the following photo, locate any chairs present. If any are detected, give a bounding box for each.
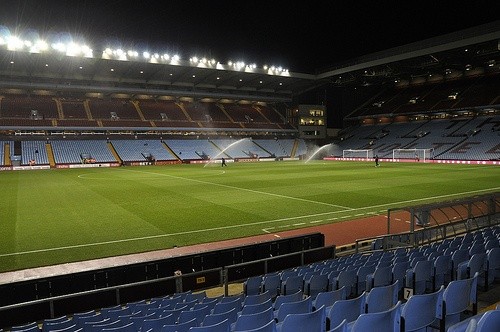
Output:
[0,70,500,168]
[0,224,500,332]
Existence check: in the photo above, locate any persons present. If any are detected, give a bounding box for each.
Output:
[222,158,226,167]
[82,158,98,163]
[29,159,37,167]
[415,153,419,162]
[374,155,380,167]
[255,154,260,163]
